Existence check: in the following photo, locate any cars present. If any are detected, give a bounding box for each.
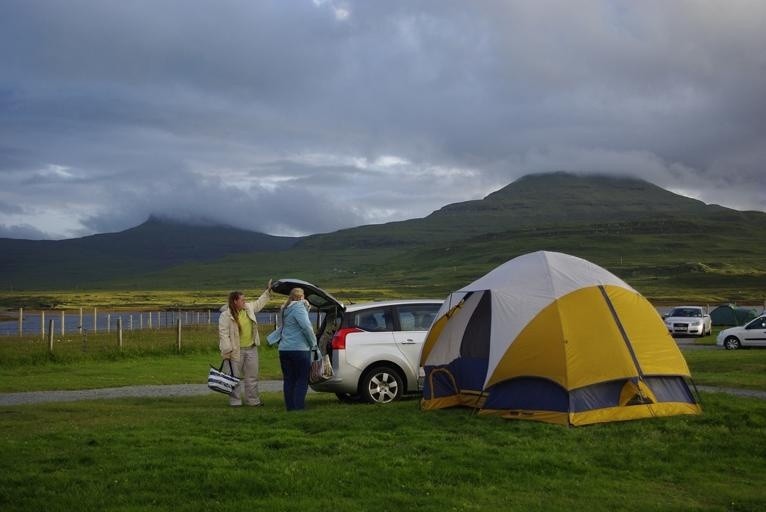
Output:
[663,305,713,337]
[715,313,765,350]
[266,279,444,405]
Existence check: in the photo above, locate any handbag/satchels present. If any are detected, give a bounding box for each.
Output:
[308,354,335,385]
[208,363,245,396]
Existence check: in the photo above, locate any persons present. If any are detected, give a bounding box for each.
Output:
[218,278,275,408]
[266,288,318,409]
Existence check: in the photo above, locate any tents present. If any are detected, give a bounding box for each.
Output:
[419,250,703,429]
